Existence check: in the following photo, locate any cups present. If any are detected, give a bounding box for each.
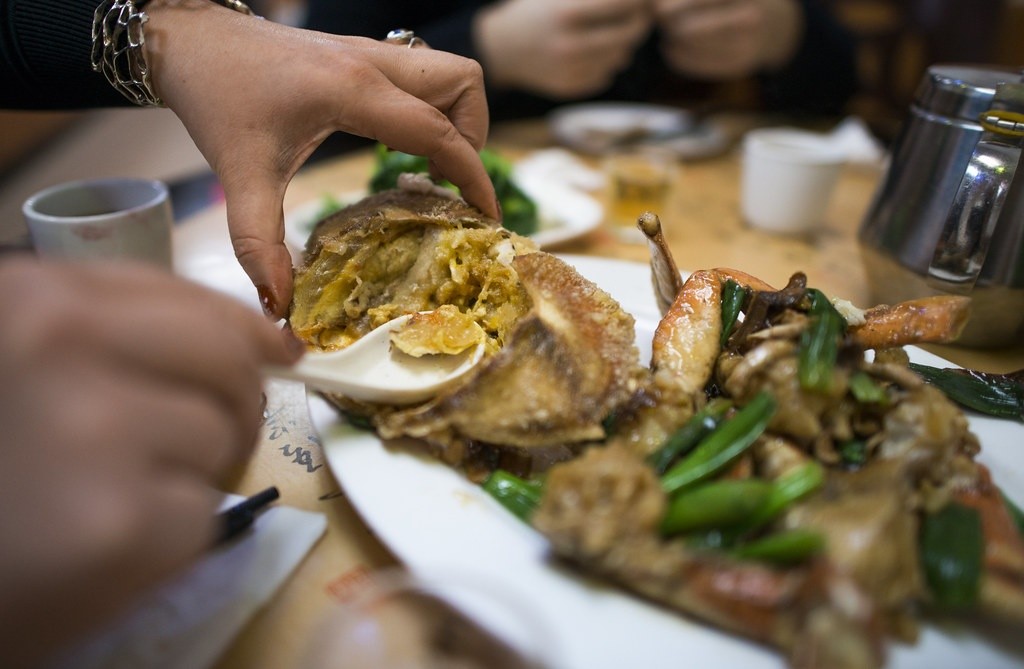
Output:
[602,146,675,241]
[742,128,839,234]
[866,68,1024,295]
[22,177,171,270]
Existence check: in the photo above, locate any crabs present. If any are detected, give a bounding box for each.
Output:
[284,170,1024,669]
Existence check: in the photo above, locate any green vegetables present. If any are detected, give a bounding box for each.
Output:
[318,141,536,238]
[485,283,1022,629]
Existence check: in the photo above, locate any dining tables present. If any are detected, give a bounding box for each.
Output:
[171,117,1024,669]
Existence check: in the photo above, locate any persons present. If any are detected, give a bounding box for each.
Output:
[1,0,518,669]
[299,1,1007,152]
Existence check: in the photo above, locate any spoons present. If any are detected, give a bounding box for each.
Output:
[274,312,484,403]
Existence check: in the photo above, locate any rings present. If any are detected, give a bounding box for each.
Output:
[383,27,433,48]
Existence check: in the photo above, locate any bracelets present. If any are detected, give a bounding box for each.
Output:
[90,1,267,110]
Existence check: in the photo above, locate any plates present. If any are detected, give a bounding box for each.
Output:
[309,256,1024,669]
[287,164,602,251]
[553,101,729,162]
